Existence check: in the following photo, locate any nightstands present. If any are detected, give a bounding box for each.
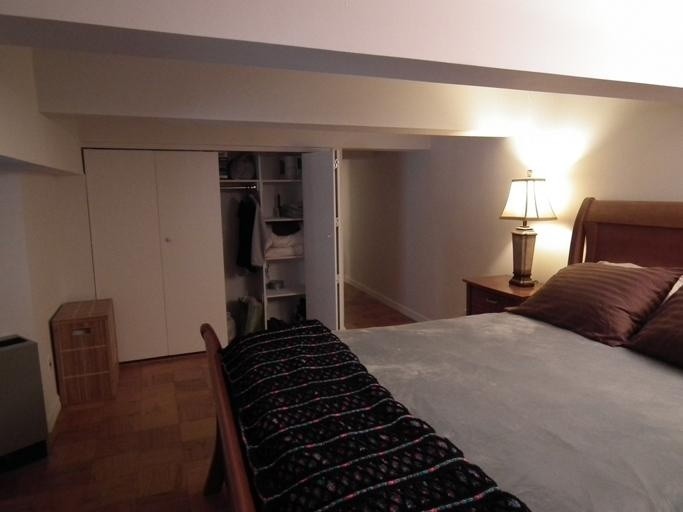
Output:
[462,275,549,316]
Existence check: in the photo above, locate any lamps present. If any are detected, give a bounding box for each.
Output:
[500,170,557,288]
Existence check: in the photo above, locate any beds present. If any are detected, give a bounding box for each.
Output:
[200,197,683,512]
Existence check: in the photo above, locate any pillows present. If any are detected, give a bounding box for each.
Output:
[504,258,683,370]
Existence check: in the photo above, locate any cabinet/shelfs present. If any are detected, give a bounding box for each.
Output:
[82,148,344,364]
[49,298,121,410]
